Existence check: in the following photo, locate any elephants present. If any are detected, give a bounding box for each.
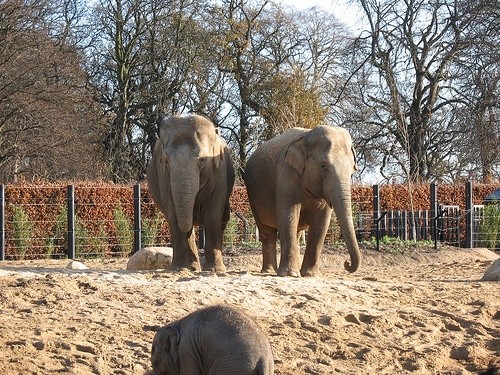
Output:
[244,125,362,278]
[147,113,235,274]
[150,304,275,375]
